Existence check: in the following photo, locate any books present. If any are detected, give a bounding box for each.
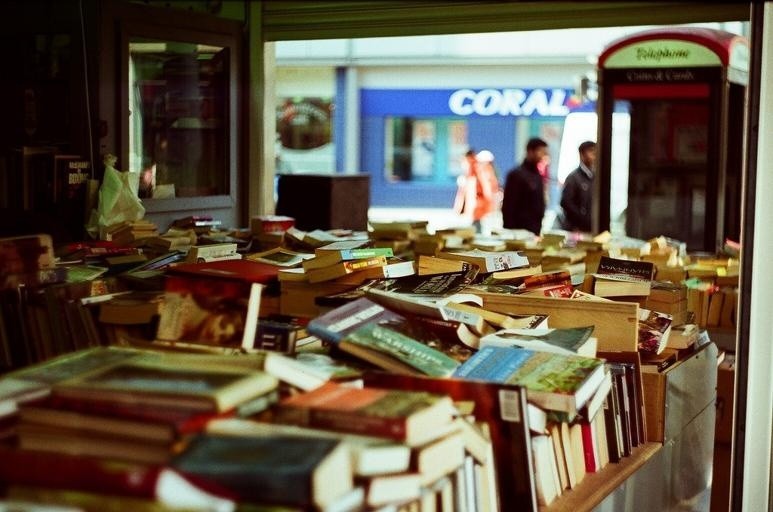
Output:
[0,137,740,512]
